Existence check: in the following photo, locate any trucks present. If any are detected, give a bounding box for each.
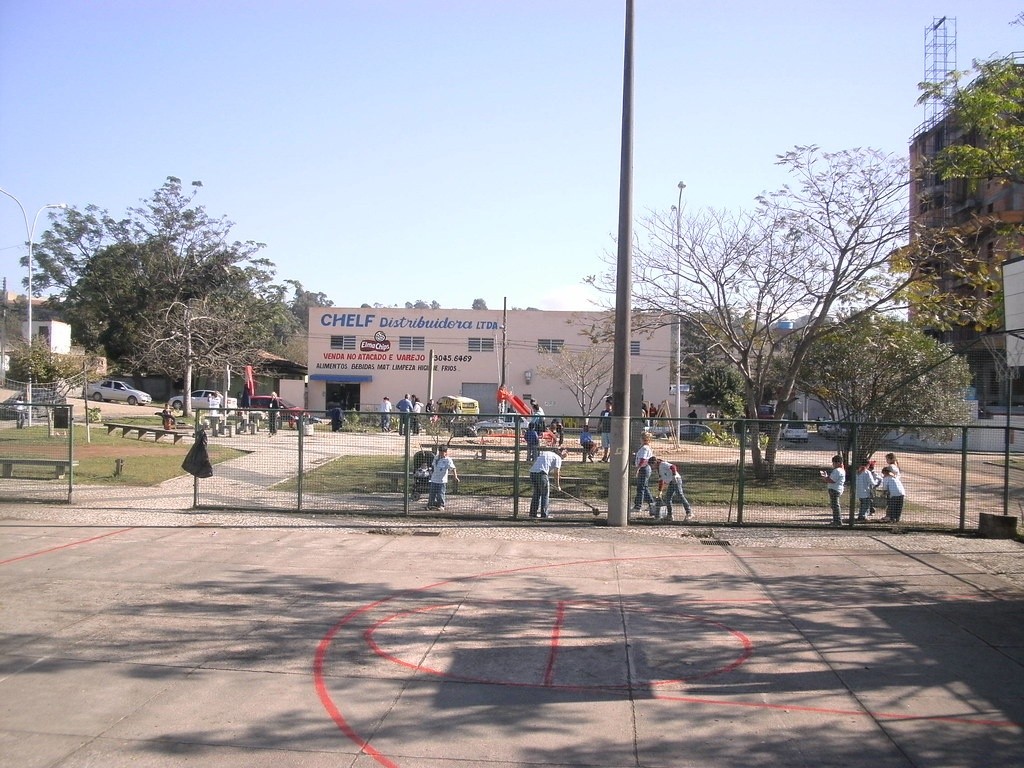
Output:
[746,404,774,419]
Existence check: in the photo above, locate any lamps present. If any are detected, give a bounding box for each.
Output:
[524,370,532,383]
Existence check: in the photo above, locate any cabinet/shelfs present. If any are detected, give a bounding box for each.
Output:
[420,443,588,463]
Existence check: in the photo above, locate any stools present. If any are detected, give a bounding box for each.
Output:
[222,424,236,437]
[244,422,257,435]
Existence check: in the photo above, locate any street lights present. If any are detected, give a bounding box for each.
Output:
[670,181,687,442]
[0,189,68,427]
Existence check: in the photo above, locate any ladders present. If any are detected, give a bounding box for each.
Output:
[649,400,679,449]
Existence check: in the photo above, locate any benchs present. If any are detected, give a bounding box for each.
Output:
[376,471,601,495]
[0,456,79,479]
[104,421,189,443]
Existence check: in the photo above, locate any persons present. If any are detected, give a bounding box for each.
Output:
[855,453,906,523]
[648,456,695,522]
[528,447,568,518]
[687,409,698,424]
[641,403,657,426]
[630,432,654,512]
[379,393,436,436]
[596,399,612,462]
[524,397,564,462]
[415,461,432,477]
[162,390,230,425]
[14,395,26,430]
[580,425,598,463]
[268,391,280,436]
[819,455,846,525]
[425,445,460,511]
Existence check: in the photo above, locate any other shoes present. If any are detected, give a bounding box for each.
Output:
[685,513,695,521]
[599,456,607,462]
[661,515,673,521]
[631,508,642,512]
[857,517,867,522]
[536,514,542,517]
[547,515,553,519]
[437,506,444,511]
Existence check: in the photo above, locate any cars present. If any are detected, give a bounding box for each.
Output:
[83,380,152,406]
[168,390,237,414]
[819,419,859,440]
[475,413,530,434]
[780,422,808,443]
[661,424,715,442]
[244,396,310,421]
[1,389,66,420]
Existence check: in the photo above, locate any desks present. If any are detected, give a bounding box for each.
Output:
[204,415,221,436]
[250,413,261,430]
[227,415,244,432]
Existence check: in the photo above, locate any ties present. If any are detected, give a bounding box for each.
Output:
[436,455,444,467]
[856,466,867,476]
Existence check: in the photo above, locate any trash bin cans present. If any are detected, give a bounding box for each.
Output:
[551,418,563,424]
[413,451,436,494]
[54,408,68,428]
[562,418,572,428]
[979,411,994,420]
[451,422,465,437]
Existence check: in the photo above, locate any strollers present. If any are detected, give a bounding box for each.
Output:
[411,451,436,502]
